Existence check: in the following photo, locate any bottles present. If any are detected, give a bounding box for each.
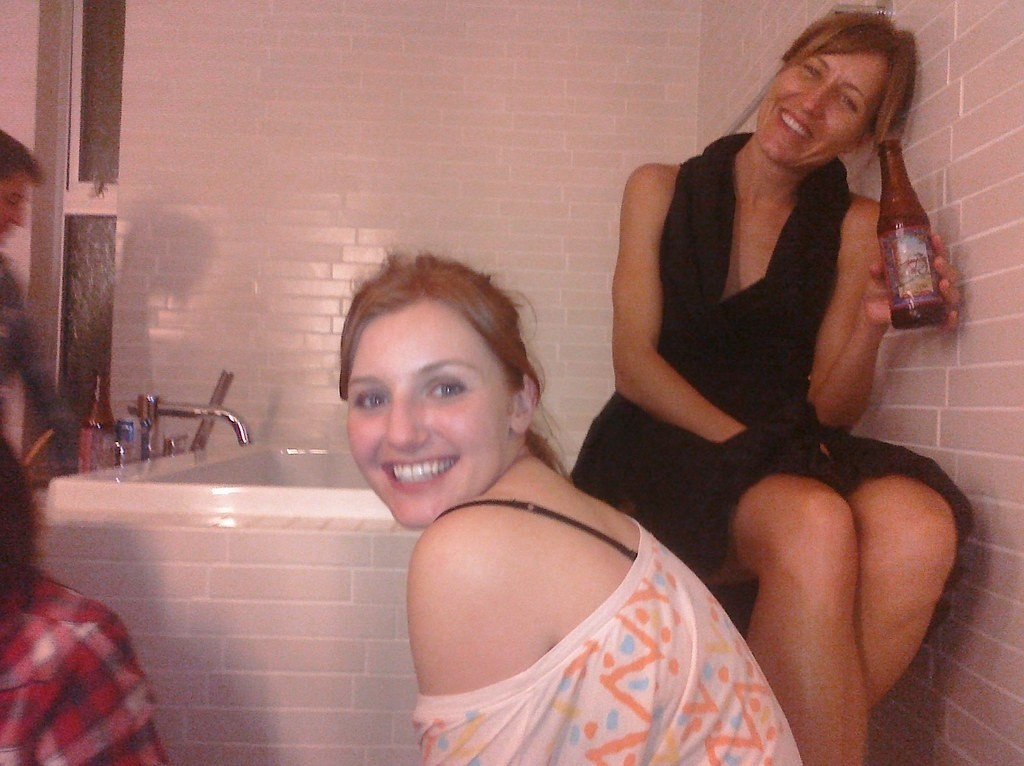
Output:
[877,141,944,328]
[78,373,117,474]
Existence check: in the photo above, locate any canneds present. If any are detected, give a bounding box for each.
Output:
[115,414,141,465]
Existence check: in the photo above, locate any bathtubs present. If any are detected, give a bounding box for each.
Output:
[45,435,582,766]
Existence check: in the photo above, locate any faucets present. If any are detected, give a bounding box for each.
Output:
[126,393,252,462]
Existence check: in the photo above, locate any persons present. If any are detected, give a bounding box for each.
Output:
[0,135,76,486]
[567,2,972,765]
[337,257,804,766]
[0,434,171,766]
